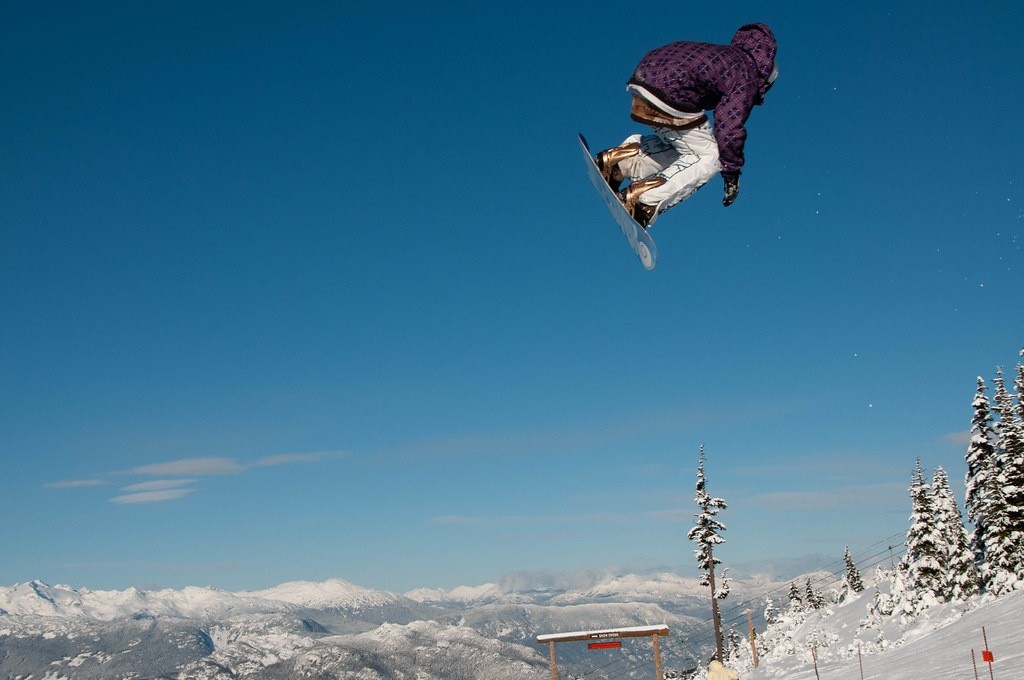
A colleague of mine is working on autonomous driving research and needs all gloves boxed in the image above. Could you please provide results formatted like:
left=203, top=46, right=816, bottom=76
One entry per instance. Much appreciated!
left=721, top=169, right=739, bottom=207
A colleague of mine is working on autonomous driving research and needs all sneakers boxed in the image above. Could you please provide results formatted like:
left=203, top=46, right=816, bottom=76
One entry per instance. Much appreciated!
left=623, top=196, right=654, bottom=232
left=605, top=150, right=626, bottom=189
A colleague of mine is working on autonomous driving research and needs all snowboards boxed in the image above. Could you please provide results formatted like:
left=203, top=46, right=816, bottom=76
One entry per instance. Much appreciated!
left=577, top=131, right=661, bottom=272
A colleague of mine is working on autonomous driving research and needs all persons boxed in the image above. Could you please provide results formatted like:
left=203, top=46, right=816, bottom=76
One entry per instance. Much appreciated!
left=594, top=22, right=778, bottom=230
